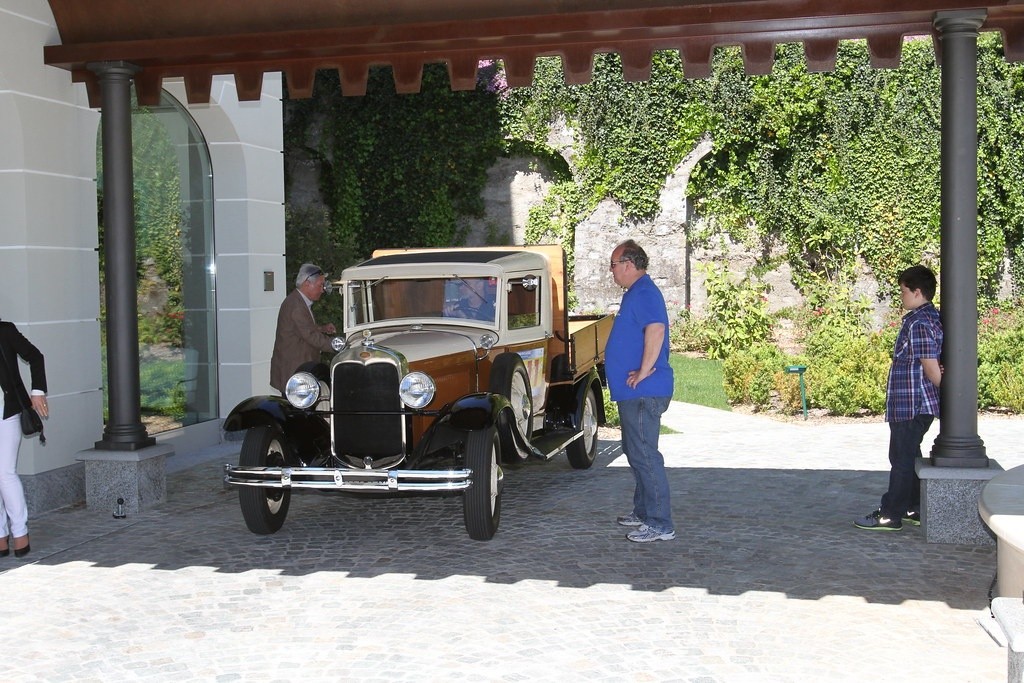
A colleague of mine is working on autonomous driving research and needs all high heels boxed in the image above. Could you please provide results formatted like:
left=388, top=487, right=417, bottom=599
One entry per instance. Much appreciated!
left=14, top=533, right=30, bottom=557
left=0, top=535, right=9, bottom=557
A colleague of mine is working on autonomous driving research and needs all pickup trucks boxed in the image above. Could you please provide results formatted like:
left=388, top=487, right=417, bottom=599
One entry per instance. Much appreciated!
left=218, top=242, right=620, bottom=543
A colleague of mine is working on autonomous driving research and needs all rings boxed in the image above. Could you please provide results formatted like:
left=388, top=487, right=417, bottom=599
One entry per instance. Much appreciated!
left=631, top=378, right=633, bottom=382
left=43, top=404, right=46, bottom=407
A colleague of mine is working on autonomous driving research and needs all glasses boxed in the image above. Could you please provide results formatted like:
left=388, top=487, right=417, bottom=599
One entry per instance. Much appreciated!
left=611, top=259, right=634, bottom=268
left=305, top=269, right=323, bottom=281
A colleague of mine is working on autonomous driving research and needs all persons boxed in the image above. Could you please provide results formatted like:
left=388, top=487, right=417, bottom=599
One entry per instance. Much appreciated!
left=270, top=263, right=339, bottom=399
left=0, top=319, right=49, bottom=558
left=440, top=277, right=495, bottom=322
left=605, top=240, right=675, bottom=543
left=853, top=265, right=944, bottom=531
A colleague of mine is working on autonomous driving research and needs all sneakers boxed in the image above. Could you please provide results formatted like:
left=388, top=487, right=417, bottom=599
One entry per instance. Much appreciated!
left=853, top=505, right=903, bottom=530
left=879, top=506, right=920, bottom=525
left=617, top=511, right=644, bottom=525
left=626, top=523, right=676, bottom=542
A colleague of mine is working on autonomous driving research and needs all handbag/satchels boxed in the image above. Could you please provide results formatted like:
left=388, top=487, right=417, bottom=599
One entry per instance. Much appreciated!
left=21, top=407, right=43, bottom=435
